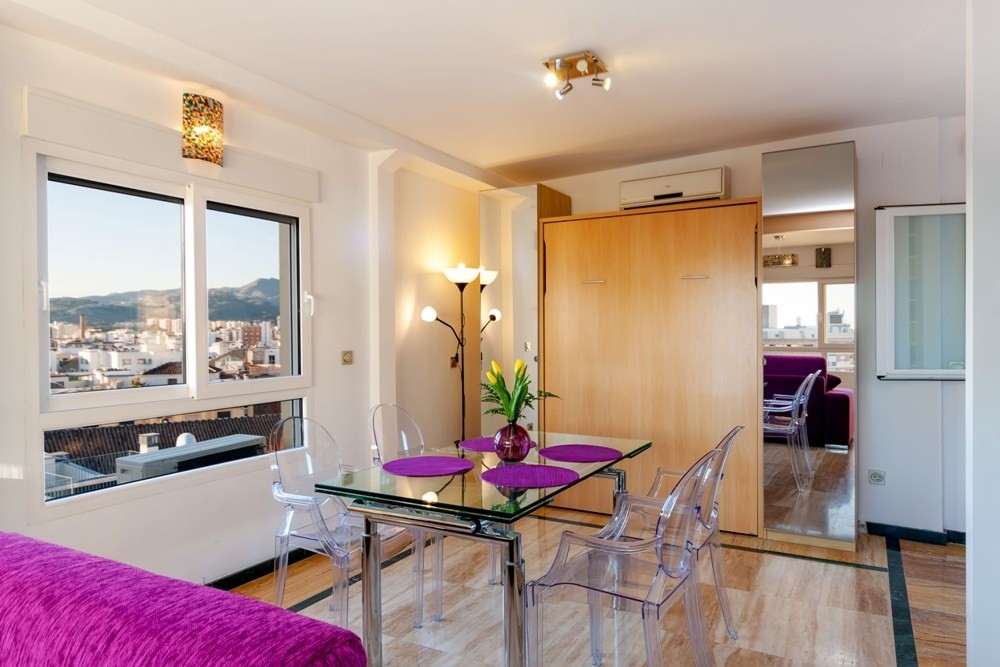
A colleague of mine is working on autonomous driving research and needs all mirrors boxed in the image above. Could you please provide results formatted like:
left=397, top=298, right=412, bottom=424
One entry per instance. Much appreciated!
left=760, top=140, right=856, bottom=546
left=482, top=451, right=540, bottom=509
left=479, top=185, right=539, bottom=430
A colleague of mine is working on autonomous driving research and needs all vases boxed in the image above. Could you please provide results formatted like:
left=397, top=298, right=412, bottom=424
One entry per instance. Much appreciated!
left=493, top=461, right=528, bottom=499
left=493, top=419, right=531, bottom=462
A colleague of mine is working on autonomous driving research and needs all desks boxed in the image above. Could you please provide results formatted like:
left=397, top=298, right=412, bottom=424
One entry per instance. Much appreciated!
left=116, top=434, right=268, bottom=485
left=44, top=449, right=144, bottom=498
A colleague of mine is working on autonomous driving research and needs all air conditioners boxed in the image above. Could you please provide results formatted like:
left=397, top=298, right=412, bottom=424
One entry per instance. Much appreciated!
left=618, top=165, right=733, bottom=211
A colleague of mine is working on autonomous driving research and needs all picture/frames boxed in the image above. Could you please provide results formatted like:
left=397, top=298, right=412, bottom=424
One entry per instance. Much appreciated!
left=872, top=202, right=966, bottom=382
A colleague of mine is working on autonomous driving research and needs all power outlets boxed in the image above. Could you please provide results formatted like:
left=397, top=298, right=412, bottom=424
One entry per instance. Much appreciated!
left=868, top=470, right=885, bottom=485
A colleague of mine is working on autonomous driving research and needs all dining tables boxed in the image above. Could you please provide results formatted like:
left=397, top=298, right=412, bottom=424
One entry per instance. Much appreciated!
left=316, top=429, right=653, bottom=667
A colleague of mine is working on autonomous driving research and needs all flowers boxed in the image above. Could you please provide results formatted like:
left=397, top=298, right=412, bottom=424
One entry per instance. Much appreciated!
left=478, top=359, right=564, bottom=422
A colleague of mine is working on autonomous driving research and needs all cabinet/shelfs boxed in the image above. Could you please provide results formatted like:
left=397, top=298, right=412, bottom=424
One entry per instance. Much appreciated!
left=760, top=140, right=856, bottom=553
left=481, top=184, right=571, bottom=431
left=539, top=195, right=763, bottom=551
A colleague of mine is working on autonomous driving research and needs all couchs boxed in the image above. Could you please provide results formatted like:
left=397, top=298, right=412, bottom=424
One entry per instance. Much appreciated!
left=0, top=532, right=368, bottom=667
left=763, top=354, right=855, bottom=449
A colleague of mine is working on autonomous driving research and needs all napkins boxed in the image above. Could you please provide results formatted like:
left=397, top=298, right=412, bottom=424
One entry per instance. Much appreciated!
left=381, top=456, right=475, bottom=476
left=459, top=436, right=536, bottom=453
left=538, top=444, right=622, bottom=463
left=480, top=464, right=580, bottom=488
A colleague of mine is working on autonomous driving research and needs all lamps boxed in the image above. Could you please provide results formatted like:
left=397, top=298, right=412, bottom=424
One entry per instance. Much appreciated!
left=421, top=444, right=466, bottom=509
left=542, top=50, right=611, bottom=99
left=479, top=270, right=502, bottom=339
left=421, top=262, right=483, bottom=440
left=182, top=93, right=224, bottom=180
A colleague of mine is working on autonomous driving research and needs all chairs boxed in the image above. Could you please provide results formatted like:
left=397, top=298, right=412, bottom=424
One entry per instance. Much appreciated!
left=763, top=368, right=822, bottom=490
left=584, top=424, right=746, bottom=655
left=523, top=446, right=723, bottom=667
left=367, top=402, right=497, bottom=622
left=269, top=415, right=429, bottom=631
